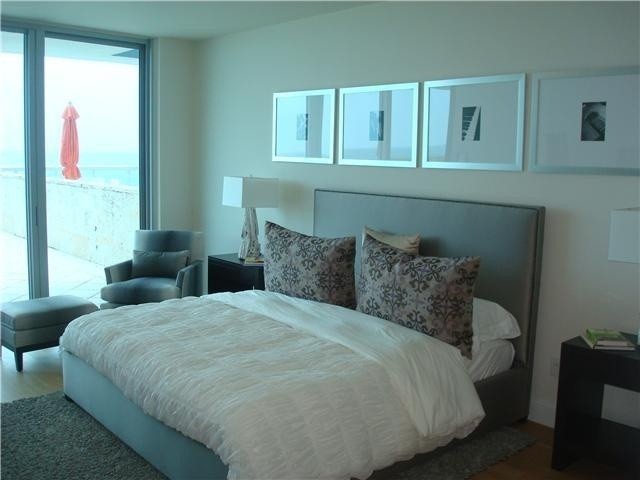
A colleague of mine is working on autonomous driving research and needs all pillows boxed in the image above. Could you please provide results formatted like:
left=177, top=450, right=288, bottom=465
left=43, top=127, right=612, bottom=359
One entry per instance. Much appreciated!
left=132, top=250, right=190, bottom=277
left=263, top=221, right=522, bottom=360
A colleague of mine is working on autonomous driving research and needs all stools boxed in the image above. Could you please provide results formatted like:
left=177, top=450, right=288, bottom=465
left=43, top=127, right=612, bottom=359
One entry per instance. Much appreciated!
left=0, top=295, right=97, bottom=372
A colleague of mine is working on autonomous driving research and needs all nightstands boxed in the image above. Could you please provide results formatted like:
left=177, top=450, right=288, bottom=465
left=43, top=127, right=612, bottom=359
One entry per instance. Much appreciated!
left=208, top=253, right=264, bottom=294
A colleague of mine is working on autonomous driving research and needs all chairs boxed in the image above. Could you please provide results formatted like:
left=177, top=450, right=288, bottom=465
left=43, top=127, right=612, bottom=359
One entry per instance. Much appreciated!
left=100, top=230, right=203, bottom=310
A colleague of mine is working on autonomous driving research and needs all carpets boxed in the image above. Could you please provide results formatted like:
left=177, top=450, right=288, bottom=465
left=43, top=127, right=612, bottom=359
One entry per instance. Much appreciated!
left=0, top=392, right=536, bottom=480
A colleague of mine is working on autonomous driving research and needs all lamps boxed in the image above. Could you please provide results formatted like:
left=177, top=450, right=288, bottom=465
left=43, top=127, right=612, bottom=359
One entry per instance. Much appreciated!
left=608, top=207, right=640, bottom=264
left=222, top=176, right=279, bottom=260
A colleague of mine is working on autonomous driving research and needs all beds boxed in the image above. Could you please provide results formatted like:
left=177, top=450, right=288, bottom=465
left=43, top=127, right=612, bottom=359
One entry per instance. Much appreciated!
left=62, top=190, right=545, bottom=480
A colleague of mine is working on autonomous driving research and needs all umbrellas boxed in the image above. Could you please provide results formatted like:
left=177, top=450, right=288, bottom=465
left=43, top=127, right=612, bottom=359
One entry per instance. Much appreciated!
left=59, top=101, right=81, bottom=180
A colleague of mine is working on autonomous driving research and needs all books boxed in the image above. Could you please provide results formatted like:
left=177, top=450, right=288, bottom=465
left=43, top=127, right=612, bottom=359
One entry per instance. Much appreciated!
left=243, top=256, right=264, bottom=268
left=578, top=328, right=634, bottom=351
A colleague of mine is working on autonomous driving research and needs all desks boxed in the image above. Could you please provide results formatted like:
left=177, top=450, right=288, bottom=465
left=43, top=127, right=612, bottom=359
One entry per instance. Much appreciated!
left=552, top=337, right=640, bottom=471
